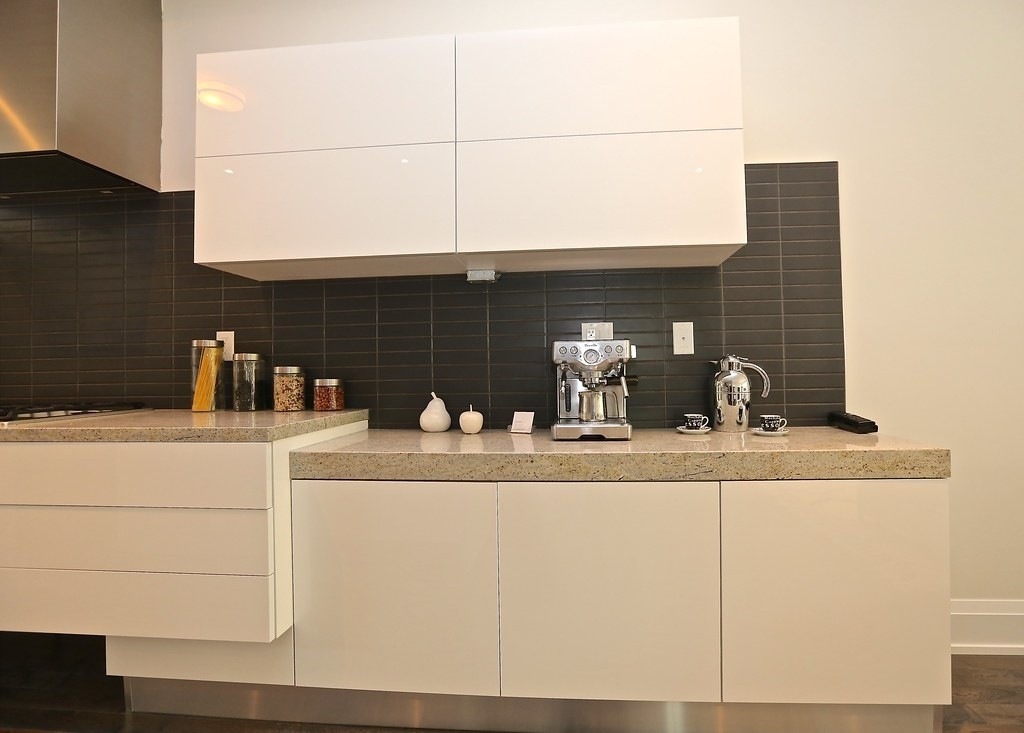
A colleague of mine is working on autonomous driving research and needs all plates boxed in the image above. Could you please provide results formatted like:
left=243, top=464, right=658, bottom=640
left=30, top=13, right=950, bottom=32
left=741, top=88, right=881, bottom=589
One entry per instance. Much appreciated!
left=751, top=428, right=789, bottom=436
left=677, top=426, right=712, bottom=434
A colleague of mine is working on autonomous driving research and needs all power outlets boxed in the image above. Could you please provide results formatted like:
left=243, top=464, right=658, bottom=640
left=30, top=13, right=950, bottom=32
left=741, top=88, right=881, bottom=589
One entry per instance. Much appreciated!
left=582, top=322, right=613, bottom=340
left=216, top=330, right=234, bottom=362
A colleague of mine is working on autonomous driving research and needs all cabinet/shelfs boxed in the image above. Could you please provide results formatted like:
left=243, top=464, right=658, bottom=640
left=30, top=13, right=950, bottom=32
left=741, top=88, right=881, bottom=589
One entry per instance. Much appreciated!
left=193, top=11, right=748, bottom=281
left=0, top=439, right=955, bottom=733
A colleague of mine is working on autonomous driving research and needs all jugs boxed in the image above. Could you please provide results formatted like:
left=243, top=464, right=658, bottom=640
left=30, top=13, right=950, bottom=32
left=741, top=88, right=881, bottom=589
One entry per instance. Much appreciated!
left=709, top=352, right=770, bottom=432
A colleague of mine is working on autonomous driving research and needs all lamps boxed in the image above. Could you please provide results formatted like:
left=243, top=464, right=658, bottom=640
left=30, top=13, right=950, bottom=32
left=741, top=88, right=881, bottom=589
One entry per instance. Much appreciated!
left=196, top=80, right=248, bottom=114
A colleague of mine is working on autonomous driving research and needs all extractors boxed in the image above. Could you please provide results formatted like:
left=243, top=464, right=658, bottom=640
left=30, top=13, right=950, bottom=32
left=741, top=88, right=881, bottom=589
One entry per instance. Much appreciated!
left=0, top=0, right=162, bottom=207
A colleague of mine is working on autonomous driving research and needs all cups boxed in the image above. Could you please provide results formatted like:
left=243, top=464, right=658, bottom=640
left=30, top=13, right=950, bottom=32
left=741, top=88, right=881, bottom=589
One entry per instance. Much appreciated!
left=760, top=415, right=787, bottom=432
left=683, top=413, right=709, bottom=430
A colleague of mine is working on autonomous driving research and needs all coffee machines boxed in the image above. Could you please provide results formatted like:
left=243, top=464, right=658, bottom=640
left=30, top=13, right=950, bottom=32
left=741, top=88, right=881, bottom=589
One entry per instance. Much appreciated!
left=552, top=339, right=632, bottom=440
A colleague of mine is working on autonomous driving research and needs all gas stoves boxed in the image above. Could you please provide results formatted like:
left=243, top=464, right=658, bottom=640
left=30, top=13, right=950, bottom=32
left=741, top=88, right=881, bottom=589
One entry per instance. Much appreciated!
left=0, top=402, right=144, bottom=421
